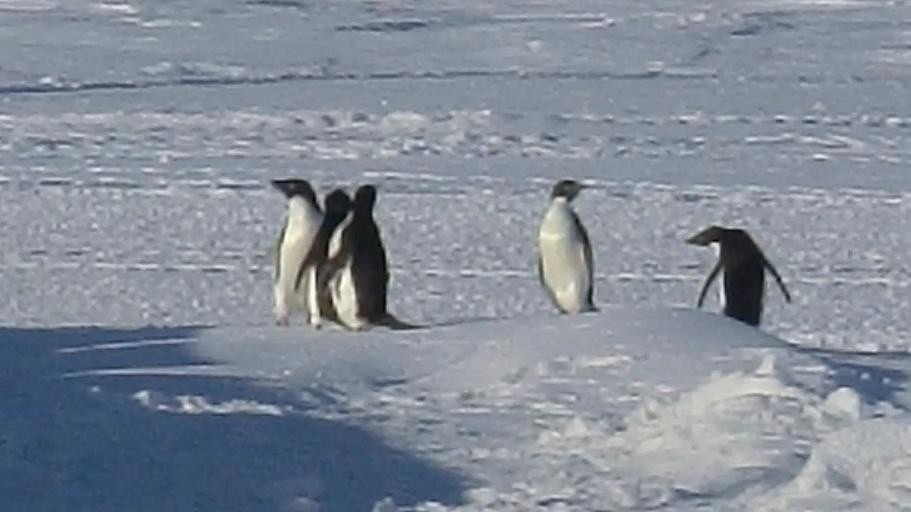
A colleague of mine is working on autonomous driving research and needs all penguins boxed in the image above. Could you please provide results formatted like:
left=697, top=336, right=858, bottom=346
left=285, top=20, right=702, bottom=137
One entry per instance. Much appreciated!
left=268, top=177, right=406, bottom=329
left=684, top=224, right=793, bottom=330
left=535, top=180, right=606, bottom=316
